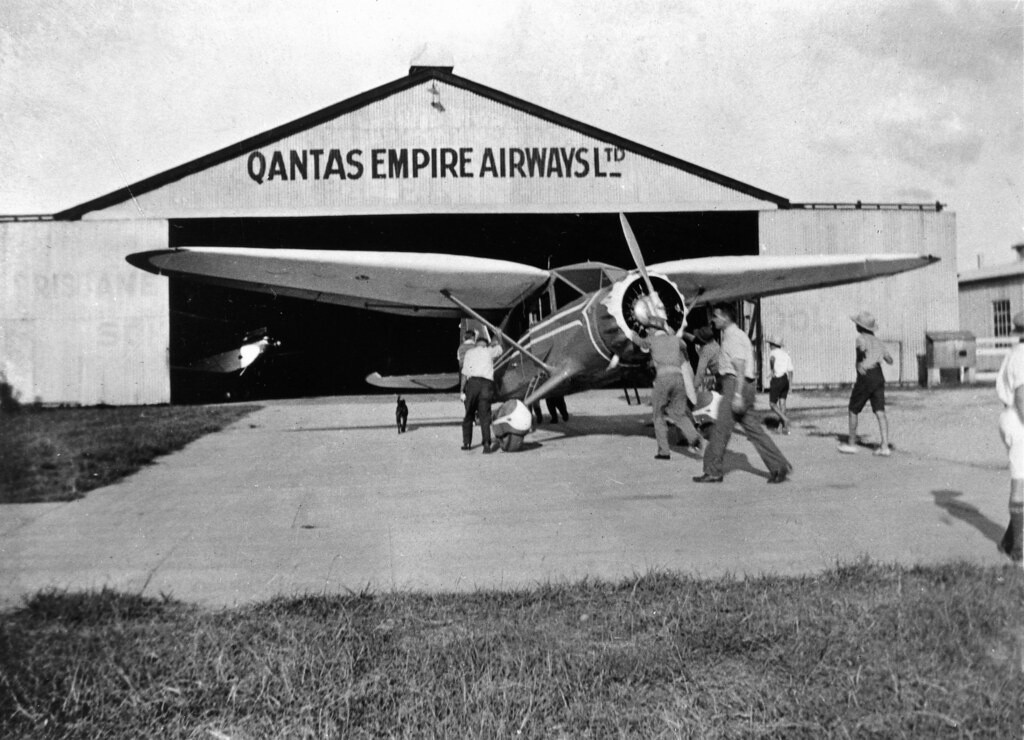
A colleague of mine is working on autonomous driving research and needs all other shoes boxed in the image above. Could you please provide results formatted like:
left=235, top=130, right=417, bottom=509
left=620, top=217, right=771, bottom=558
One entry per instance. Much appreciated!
left=767, top=463, right=793, bottom=484
left=775, top=421, right=792, bottom=436
left=872, top=447, right=891, bottom=456
left=692, top=474, right=723, bottom=484
left=461, top=443, right=471, bottom=451
left=690, top=437, right=703, bottom=451
left=838, top=443, right=859, bottom=454
left=654, top=453, right=670, bottom=459
left=483, top=445, right=490, bottom=453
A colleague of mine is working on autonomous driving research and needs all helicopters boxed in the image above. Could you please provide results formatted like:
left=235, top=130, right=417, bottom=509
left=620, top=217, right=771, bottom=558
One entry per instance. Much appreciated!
left=124, top=213, right=944, bottom=452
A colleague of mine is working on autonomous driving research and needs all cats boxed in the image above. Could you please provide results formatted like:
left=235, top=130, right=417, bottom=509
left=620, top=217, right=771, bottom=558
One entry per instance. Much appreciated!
left=396, top=395, right=408, bottom=434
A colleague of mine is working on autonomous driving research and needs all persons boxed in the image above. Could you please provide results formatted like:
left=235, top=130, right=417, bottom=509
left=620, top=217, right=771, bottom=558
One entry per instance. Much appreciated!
left=633, top=316, right=703, bottom=460
left=995, top=310, right=1024, bottom=563
left=528, top=400, right=543, bottom=432
left=764, top=333, right=793, bottom=436
left=460, top=337, right=503, bottom=453
left=683, top=327, right=726, bottom=396
left=837, top=310, right=892, bottom=456
left=692, top=300, right=793, bottom=484
left=545, top=396, right=569, bottom=425
left=457, top=330, right=481, bottom=425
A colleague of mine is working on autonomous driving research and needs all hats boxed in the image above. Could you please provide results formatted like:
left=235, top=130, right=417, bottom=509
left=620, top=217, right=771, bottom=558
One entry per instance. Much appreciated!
left=765, top=335, right=784, bottom=347
left=850, top=311, right=878, bottom=332
left=643, top=316, right=666, bottom=330
left=693, top=326, right=714, bottom=344
left=1009, top=310, right=1024, bottom=339
